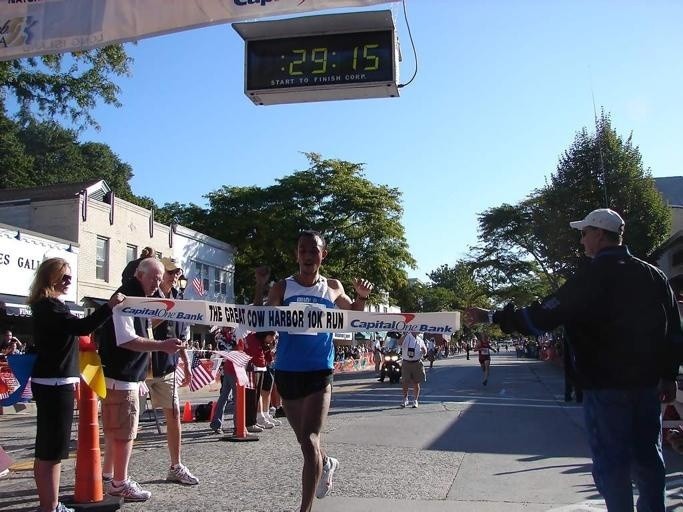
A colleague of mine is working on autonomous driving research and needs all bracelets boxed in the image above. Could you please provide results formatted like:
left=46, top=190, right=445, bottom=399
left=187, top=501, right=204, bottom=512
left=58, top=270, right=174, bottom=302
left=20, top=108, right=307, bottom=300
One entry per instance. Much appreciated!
left=488, top=309, right=496, bottom=324
left=357, top=294, right=370, bottom=301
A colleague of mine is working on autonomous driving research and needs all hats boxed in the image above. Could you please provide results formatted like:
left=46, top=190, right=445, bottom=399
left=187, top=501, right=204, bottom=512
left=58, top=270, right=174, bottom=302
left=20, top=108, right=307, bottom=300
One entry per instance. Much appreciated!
left=569, top=209, right=625, bottom=236
left=160, top=257, right=183, bottom=275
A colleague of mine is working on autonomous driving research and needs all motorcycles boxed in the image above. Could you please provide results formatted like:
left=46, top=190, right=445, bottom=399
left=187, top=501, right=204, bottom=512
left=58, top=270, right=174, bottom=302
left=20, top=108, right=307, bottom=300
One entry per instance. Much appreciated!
left=376, top=347, right=403, bottom=384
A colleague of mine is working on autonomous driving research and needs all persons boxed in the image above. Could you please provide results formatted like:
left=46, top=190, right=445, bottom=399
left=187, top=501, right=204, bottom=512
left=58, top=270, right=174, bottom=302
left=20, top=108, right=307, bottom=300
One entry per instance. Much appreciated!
left=122, top=246, right=157, bottom=284
left=29, top=257, right=126, bottom=512
left=254, top=230, right=374, bottom=512
left=1, top=330, right=26, bottom=357
left=334, top=331, right=565, bottom=386
left=467, top=207, right=683, bottom=511
left=93, top=258, right=187, bottom=501
left=205, top=327, right=282, bottom=434
left=181, top=335, right=212, bottom=359
left=398, top=332, right=428, bottom=408
left=101, top=254, right=200, bottom=486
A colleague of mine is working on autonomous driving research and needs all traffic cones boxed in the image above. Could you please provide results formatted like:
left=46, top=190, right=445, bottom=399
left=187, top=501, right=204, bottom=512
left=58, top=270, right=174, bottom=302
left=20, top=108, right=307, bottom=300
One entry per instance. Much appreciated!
left=54, top=342, right=124, bottom=511
left=661, top=405, right=682, bottom=446
left=208, top=400, right=217, bottom=423
left=180, top=401, right=195, bottom=423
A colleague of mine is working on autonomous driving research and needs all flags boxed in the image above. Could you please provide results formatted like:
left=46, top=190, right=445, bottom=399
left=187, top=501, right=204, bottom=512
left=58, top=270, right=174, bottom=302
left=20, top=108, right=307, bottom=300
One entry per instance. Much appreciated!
left=188, top=354, right=214, bottom=392
left=193, top=272, right=206, bottom=296
left=215, top=350, right=252, bottom=366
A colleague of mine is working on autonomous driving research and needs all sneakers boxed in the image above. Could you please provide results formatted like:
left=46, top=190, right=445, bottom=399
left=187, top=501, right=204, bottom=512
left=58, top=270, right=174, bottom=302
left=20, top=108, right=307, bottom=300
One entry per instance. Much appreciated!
left=315, top=457, right=339, bottom=498
left=412, top=400, right=418, bottom=407
left=37, top=407, right=286, bottom=512
left=400, top=398, right=409, bottom=407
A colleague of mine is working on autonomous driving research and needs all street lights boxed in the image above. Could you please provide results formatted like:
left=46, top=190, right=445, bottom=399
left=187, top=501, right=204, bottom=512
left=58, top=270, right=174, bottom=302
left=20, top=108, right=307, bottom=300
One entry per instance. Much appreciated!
left=177, top=275, right=187, bottom=301
